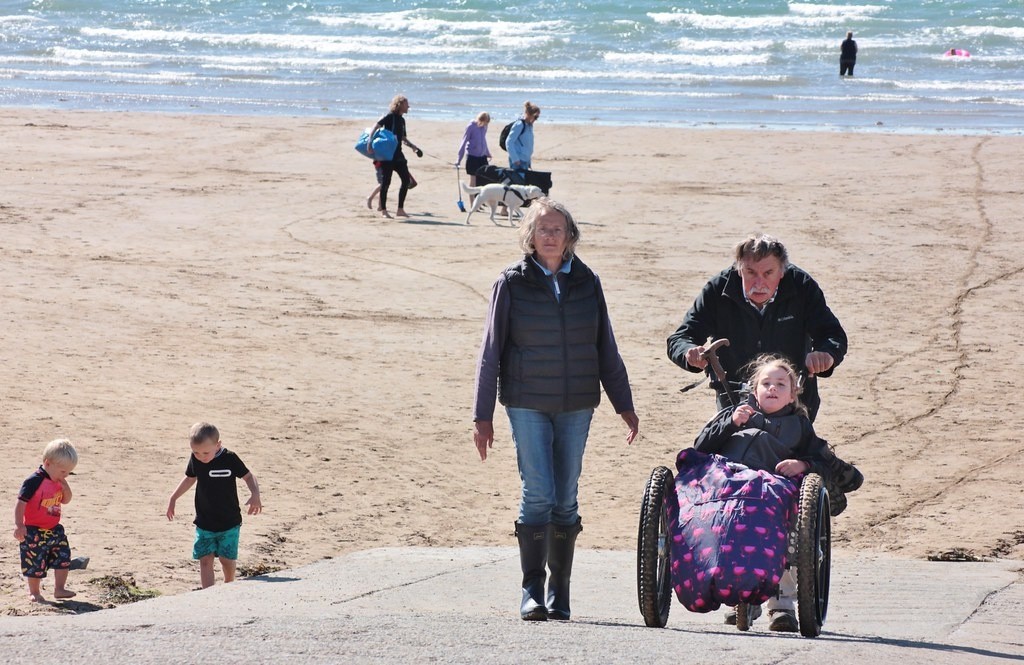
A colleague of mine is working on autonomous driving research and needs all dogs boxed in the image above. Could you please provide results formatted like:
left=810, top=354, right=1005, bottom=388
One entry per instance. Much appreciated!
left=461, top=182, right=545, bottom=226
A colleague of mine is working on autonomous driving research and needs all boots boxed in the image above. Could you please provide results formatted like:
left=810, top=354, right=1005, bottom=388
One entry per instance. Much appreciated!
left=547, top=514, right=583, bottom=619
left=514, top=519, right=550, bottom=621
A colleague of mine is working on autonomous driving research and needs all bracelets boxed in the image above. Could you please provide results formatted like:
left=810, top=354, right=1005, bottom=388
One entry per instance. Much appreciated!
left=368, top=140, right=372, bottom=142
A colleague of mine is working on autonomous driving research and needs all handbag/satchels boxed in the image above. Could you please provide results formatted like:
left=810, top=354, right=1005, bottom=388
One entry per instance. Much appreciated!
left=355, top=112, right=398, bottom=161
left=475, top=165, right=552, bottom=206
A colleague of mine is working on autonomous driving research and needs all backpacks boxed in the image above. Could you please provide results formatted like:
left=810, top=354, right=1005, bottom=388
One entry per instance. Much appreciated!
left=499, top=118, right=525, bottom=152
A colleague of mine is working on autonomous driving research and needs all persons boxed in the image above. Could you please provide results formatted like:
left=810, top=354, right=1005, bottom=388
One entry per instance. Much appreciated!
left=693, top=356, right=832, bottom=478
left=472, top=197, right=639, bottom=620
left=367, top=96, right=423, bottom=218
left=455, top=112, right=491, bottom=211
left=667, top=234, right=848, bottom=632
left=13, top=439, right=78, bottom=602
left=166, top=422, right=262, bottom=589
left=839, top=31, right=858, bottom=76
left=500, top=102, right=540, bottom=215
left=367, top=160, right=383, bottom=211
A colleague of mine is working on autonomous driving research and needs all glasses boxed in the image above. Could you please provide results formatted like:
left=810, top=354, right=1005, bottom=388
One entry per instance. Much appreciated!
left=533, top=114, right=539, bottom=120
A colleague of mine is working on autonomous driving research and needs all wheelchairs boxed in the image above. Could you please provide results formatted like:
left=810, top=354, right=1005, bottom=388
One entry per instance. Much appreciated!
left=632, top=337, right=834, bottom=640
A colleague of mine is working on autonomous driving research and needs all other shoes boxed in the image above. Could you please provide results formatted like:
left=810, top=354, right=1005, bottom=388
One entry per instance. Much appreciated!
left=768, top=610, right=799, bottom=632
left=725, top=604, right=763, bottom=625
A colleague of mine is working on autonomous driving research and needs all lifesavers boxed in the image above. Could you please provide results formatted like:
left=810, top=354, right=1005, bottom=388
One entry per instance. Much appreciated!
left=943, top=47, right=970, bottom=57
